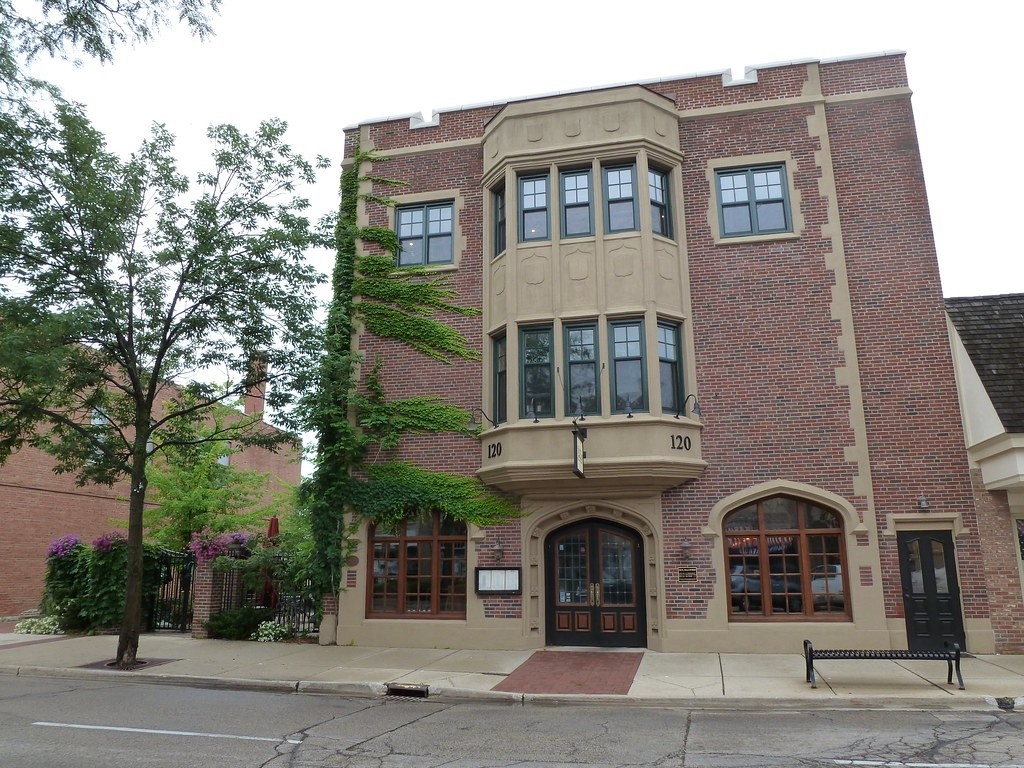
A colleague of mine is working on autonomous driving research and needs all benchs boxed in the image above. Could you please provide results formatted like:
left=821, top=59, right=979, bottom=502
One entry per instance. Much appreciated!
left=803, top=640, right=966, bottom=690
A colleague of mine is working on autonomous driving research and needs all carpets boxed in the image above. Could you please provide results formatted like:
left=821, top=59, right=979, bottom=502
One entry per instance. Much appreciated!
left=487, top=651, right=646, bottom=695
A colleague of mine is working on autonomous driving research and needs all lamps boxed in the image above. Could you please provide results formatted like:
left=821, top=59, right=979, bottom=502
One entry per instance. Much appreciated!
left=468, top=394, right=703, bottom=429
left=681, top=537, right=694, bottom=560
left=492, top=537, right=504, bottom=562
left=917, top=492, right=929, bottom=510
